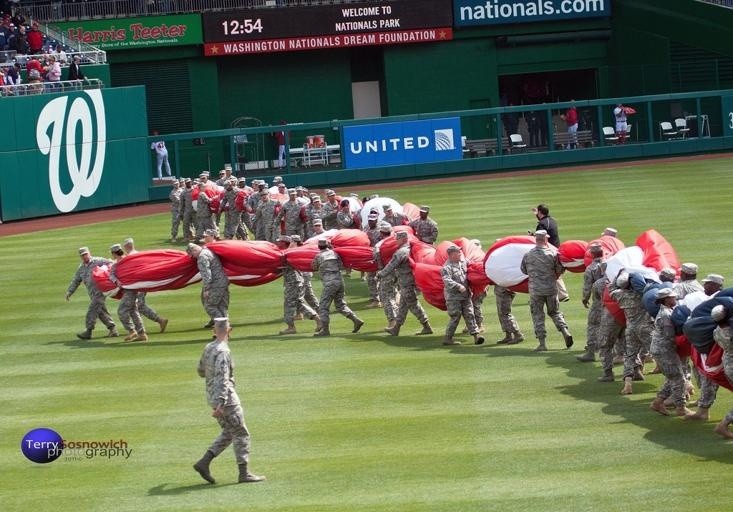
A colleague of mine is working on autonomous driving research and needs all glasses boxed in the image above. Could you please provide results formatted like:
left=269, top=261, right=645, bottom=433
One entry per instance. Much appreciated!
left=219, top=172, right=225, bottom=175
left=278, top=186, right=286, bottom=189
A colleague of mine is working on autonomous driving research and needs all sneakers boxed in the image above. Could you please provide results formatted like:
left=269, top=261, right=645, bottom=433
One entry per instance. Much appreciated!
left=559, top=295, right=569, bottom=302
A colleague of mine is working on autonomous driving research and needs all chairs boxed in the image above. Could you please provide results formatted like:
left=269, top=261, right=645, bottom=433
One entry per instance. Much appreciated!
left=660, top=121, right=678, bottom=140
left=623, top=125, right=632, bottom=144
left=510, top=134, right=527, bottom=155
left=602, top=126, right=620, bottom=147
left=675, top=118, right=690, bottom=140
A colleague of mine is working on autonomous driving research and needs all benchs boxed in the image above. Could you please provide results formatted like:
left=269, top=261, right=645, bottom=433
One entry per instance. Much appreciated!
left=465, top=137, right=512, bottom=157
left=553, top=130, right=593, bottom=150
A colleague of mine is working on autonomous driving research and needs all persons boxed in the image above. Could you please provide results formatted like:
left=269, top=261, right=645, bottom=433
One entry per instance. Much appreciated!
left=186, top=240, right=231, bottom=338
left=364, top=214, right=393, bottom=309
left=409, top=207, right=438, bottom=245
left=373, top=228, right=436, bottom=337
left=582, top=223, right=733, bottom=442
left=500, top=101, right=523, bottom=152
left=289, top=233, right=321, bottom=323
left=311, top=239, right=365, bottom=338
left=528, top=202, right=570, bottom=301
left=441, top=243, right=484, bottom=347
left=526, top=101, right=543, bottom=148
left=109, top=244, right=149, bottom=344
left=188, top=317, right=267, bottom=485
left=519, top=230, right=576, bottom=351
left=64, top=246, right=121, bottom=341
left=381, top=203, right=410, bottom=229
left=1, top=1, right=82, bottom=95
left=171, top=167, right=375, bottom=245
left=276, top=234, right=322, bottom=336
left=559, top=95, right=585, bottom=151
left=373, top=220, right=403, bottom=333
left=123, top=237, right=169, bottom=335
left=269, top=120, right=293, bottom=169
left=614, top=97, right=632, bottom=148
left=493, top=237, right=526, bottom=346
left=149, top=131, right=173, bottom=178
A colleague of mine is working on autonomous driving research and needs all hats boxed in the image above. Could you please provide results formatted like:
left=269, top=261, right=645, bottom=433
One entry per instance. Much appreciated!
left=226, top=167, right=233, bottom=171
left=657, top=289, right=677, bottom=299
left=702, top=274, right=726, bottom=287
left=172, top=180, right=180, bottom=185
left=287, top=189, right=298, bottom=195
left=617, top=272, right=629, bottom=288
left=256, top=180, right=265, bottom=185
left=534, top=229, right=550, bottom=238
left=471, top=239, right=482, bottom=249
left=446, top=246, right=460, bottom=253
left=326, top=190, right=336, bottom=197
left=198, top=182, right=207, bottom=189
left=79, top=247, right=89, bottom=255
left=318, top=237, right=327, bottom=246
left=312, top=218, right=322, bottom=226
left=310, top=192, right=316, bottom=197
left=200, top=174, right=206, bottom=177
left=180, top=178, right=185, bottom=182
left=212, top=318, right=230, bottom=328
left=110, top=244, right=122, bottom=252
left=370, top=194, right=378, bottom=199
left=495, top=239, right=501, bottom=243
left=312, top=195, right=322, bottom=203
left=351, top=193, right=359, bottom=200
left=659, top=268, right=677, bottom=280
left=203, top=171, right=209, bottom=176
left=184, top=178, right=191, bottom=183
left=680, top=262, right=698, bottom=275
left=711, top=305, right=725, bottom=322
left=601, top=227, right=618, bottom=239
left=230, top=178, right=236, bottom=182
left=259, top=183, right=268, bottom=188
left=239, top=177, right=245, bottom=183
left=296, top=186, right=302, bottom=191
left=259, top=189, right=269, bottom=195
left=291, top=235, right=301, bottom=241
left=370, top=207, right=380, bottom=213
left=253, top=180, right=257, bottom=185
left=123, top=238, right=134, bottom=247
left=194, top=179, right=200, bottom=183
left=219, top=170, right=226, bottom=174
left=396, top=231, right=408, bottom=240
left=367, top=214, right=379, bottom=221
left=382, top=203, right=392, bottom=212
left=303, top=188, right=308, bottom=192
left=419, top=206, right=430, bottom=213
left=223, top=180, right=231, bottom=190
left=379, top=221, right=391, bottom=232
left=589, top=244, right=604, bottom=253
left=273, top=177, right=282, bottom=182
left=276, top=236, right=290, bottom=243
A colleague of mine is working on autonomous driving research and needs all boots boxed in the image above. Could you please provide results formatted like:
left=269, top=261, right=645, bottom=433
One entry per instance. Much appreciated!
left=182, top=237, right=188, bottom=242
left=686, top=405, right=710, bottom=421
left=612, top=354, right=625, bottom=364
left=293, top=311, right=304, bottom=321
left=560, top=327, right=575, bottom=349
left=125, top=329, right=138, bottom=341
left=474, top=333, right=486, bottom=345
left=479, top=326, right=485, bottom=333
left=349, top=314, right=365, bottom=333
left=665, top=396, right=681, bottom=405
left=685, top=380, right=694, bottom=393
left=716, top=418, right=732, bottom=439
left=443, top=335, right=461, bottom=345
left=463, top=326, right=468, bottom=333
left=171, top=234, right=176, bottom=243
left=684, top=393, right=690, bottom=399
left=77, top=330, right=92, bottom=340
left=497, top=332, right=513, bottom=344
left=649, top=398, right=670, bottom=416
left=346, top=269, right=352, bottom=277
left=509, top=331, right=524, bottom=345
left=193, top=450, right=222, bottom=486
left=366, top=299, right=381, bottom=308
left=106, top=327, right=118, bottom=337
left=634, top=367, right=644, bottom=379
left=416, top=321, right=434, bottom=335
left=644, top=355, right=654, bottom=362
left=577, top=350, right=596, bottom=363
left=676, top=406, right=695, bottom=416
left=599, top=371, right=614, bottom=381
left=313, top=315, right=324, bottom=331
left=620, top=376, right=633, bottom=395
left=395, top=293, right=401, bottom=305
left=134, top=333, right=147, bottom=342
left=360, top=272, right=365, bottom=281
left=533, top=338, right=548, bottom=352
left=159, top=318, right=169, bottom=333
left=649, top=366, right=661, bottom=374
left=384, top=322, right=402, bottom=336
left=194, top=237, right=201, bottom=243
left=388, top=320, right=395, bottom=327
left=236, top=462, right=268, bottom=484
left=313, top=322, right=331, bottom=337
left=280, top=322, right=297, bottom=334
